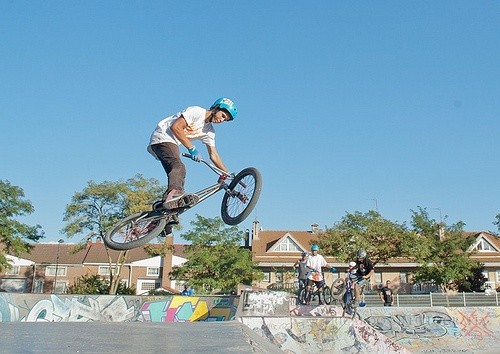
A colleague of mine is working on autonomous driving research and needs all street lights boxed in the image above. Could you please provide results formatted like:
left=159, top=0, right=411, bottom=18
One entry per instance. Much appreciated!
left=53, top=238, right=65, bottom=294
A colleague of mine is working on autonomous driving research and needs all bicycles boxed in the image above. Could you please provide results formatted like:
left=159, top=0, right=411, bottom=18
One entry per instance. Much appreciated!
left=297, top=268, right=333, bottom=306
left=104, top=153, right=263, bottom=251
left=331, top=268, right=365, bottom=320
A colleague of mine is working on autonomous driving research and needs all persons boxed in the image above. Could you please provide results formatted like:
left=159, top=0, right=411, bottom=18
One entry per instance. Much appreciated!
left=146, top=97, right=239, bottom=235
left=346, top=248, right=375, bottom=307
left=293, top=245, right=336, bottom=305
left=379, top=280, right=395, bottom=306
